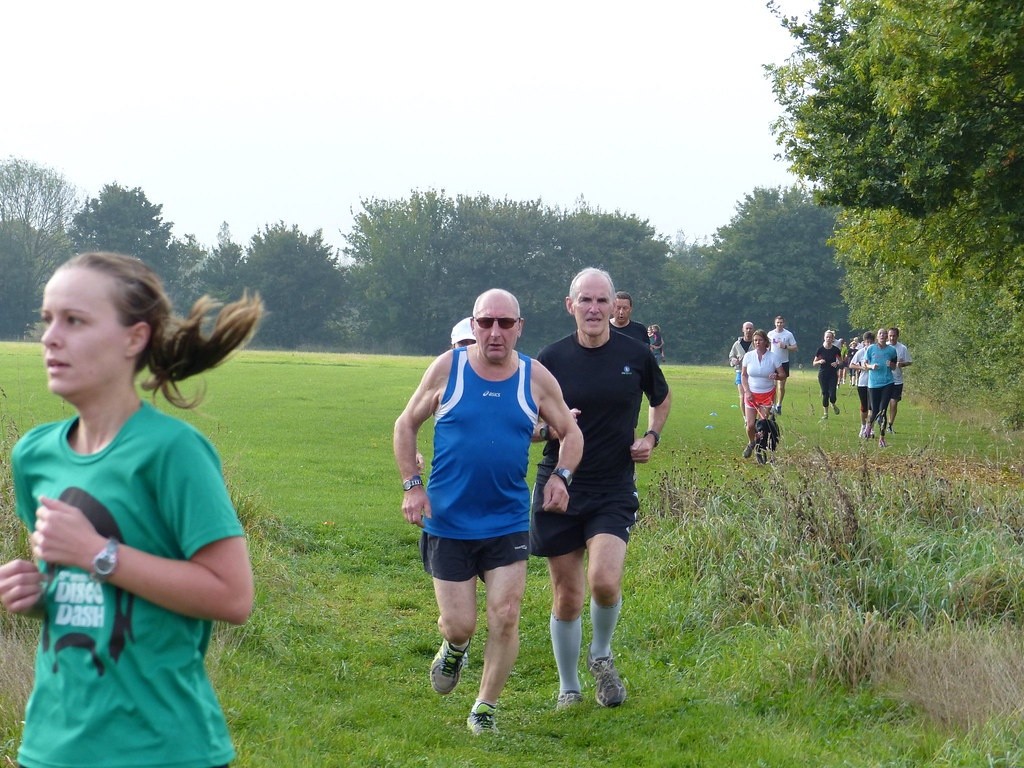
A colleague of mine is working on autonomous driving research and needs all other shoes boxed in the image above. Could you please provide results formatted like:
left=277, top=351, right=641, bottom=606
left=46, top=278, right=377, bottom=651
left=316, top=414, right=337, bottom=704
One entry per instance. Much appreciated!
left=879, top=440, right=886, bottom=448
left=864, top=425, right=870, bottom=438
left=743, top=422, right=747, bottom=427
left=889, top=426, right=892, bottom=432
left=822, top=414, right=828, bottom=419
left=776, top=405, right=781, bottom=414
left=859, top=427, right=865, bottom=437
left=871, top=428, right=875, bottom=435
left=831, top=405, right=839, bottom=414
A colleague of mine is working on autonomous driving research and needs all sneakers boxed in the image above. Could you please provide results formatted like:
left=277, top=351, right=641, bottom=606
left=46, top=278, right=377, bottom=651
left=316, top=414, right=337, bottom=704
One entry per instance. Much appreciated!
left=743, top=442, right=755, bottom=457
left=586, top=643, right=626, bottom=707
left=430, top=636, right=471, bottom=694
left=557, top=690, right=582, bottom=711
left=467, top=702, right=500, bottom=736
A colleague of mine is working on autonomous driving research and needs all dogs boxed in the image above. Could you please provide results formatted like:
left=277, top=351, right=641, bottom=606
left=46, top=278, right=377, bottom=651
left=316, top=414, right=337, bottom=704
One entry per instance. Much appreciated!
left=755, top=412, right=781, bottom=463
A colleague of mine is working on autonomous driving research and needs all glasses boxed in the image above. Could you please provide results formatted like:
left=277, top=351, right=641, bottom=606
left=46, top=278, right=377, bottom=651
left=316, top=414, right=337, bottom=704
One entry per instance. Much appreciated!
left=472, top=316, right=521, bottom=329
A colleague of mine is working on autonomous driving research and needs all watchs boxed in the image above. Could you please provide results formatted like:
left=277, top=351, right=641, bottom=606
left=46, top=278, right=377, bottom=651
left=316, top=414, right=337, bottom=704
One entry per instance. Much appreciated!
left=403, top=478, right=422, bottom=491
left=89, top=536, right=120, bottom=584
left=540, top=424, right=550, bottom=441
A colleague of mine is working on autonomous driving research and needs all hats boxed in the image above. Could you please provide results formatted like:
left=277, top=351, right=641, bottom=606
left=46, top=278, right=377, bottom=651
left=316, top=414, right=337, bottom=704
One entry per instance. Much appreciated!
left=451, top=317, right=477, bottom=344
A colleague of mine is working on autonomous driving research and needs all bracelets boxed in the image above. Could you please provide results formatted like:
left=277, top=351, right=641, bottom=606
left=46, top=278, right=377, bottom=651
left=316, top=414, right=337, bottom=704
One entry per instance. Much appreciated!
left=783, top=345, right=787, bottom=349
left=552, top=468, right=573, bottom=486
left=403, top=475, right=420, bottom=483
left=644, top=430, right=661, bottom=447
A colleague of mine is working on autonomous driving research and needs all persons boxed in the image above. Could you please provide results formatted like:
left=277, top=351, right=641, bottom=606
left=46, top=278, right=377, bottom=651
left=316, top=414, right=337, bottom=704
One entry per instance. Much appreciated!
left=741, top=329, right=787, bottom=462
left=646, top=324, right=666, bottom=367
left=530, top=266, right=672, bottom=709
left=729, top=322, right=757, bottom=425
left=608, top=289, right=650, bottom=345
left=813, top=328, right=847, bottom=419
left=394, top=288, right=584, bottom=735
left=1, top=250, right=268, bottom=768
left=846, top=328, right=913, bottom=447
left=767, top=315, right=799, bottom=415
left=449, top=319, right=489, bottom=670
